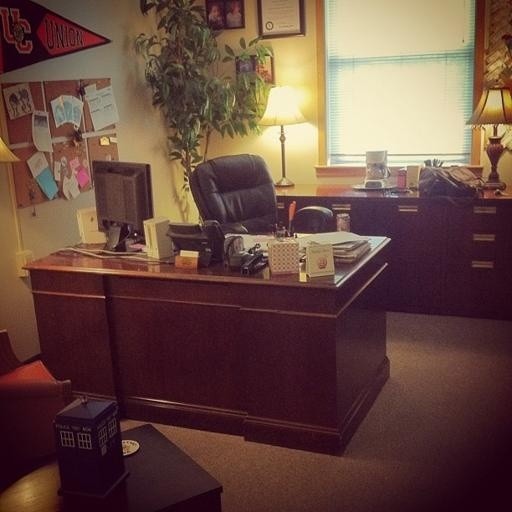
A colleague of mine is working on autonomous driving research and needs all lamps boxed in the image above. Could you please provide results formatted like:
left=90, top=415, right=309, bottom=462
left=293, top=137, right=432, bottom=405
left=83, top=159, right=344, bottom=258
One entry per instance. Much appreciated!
left=466, top=87, right=512, bottom=190
left=257, top=85, right=310, bottom=188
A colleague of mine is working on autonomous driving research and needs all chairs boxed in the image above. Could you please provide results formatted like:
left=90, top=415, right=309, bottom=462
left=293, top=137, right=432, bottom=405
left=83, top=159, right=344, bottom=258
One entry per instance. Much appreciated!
left=0, top=329, right=74, bottom=480
left=188, top=154, right=334, bottom=239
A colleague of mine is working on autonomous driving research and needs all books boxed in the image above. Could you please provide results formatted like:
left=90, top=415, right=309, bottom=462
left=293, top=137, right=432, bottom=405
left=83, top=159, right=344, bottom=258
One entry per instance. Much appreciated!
left=294, top=230, right=373, bottom=263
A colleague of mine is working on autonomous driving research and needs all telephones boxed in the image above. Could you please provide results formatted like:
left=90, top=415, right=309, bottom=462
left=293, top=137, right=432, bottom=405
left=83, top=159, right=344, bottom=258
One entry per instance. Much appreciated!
left=167, top=219, right=227, bottom=266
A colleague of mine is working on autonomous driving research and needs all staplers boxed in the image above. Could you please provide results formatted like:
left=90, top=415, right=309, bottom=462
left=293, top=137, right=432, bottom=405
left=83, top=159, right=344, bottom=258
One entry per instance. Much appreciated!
left=240, top=253, right=267, bottom=275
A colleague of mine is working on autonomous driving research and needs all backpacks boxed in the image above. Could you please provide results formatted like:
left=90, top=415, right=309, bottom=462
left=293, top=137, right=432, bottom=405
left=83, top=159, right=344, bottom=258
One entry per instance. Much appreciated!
left=419, top=167, right=484, bottom=196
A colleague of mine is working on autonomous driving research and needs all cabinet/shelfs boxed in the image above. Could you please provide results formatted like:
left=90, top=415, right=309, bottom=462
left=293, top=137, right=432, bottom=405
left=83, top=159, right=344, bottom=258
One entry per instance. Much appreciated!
left=263, top=184, right=512, bottom=323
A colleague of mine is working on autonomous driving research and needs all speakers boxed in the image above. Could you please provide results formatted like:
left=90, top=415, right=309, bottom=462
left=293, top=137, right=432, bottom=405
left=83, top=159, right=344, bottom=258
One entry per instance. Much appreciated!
left=142, top=216, right=174, bottom=260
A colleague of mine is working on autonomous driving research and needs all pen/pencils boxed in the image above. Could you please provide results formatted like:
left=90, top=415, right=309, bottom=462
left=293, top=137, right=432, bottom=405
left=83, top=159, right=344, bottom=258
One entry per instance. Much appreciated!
left=288, top=201, right=298, bottom=237
left=424, top=159, right=444, bottom=167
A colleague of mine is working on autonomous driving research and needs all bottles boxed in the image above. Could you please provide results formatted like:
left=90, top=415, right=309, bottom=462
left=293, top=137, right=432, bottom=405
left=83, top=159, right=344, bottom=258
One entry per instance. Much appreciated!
left=396, top=168, right=407, bottom=193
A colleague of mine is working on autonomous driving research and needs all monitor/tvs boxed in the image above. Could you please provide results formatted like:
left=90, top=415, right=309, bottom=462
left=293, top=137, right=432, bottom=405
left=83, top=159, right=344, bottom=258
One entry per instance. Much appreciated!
left=92, top=160, right=154, bottom=257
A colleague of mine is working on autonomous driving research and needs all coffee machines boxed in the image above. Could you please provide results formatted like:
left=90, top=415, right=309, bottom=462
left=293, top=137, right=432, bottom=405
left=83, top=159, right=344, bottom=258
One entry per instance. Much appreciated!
left=363, top=150, right=392, bottom=189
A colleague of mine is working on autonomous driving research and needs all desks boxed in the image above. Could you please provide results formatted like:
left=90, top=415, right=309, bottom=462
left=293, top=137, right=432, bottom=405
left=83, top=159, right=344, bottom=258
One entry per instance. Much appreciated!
left=22, top=233, right=392, bottom=458
left=0, top=423, right=228, bottom=512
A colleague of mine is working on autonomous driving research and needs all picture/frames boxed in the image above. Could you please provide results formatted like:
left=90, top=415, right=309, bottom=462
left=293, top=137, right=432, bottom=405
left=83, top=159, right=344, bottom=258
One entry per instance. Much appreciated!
left=235, top=55, right=275, bottom=88
left=257, top=0, right=306, bottom=40
left=206, top=0, right=246, bottom=30
